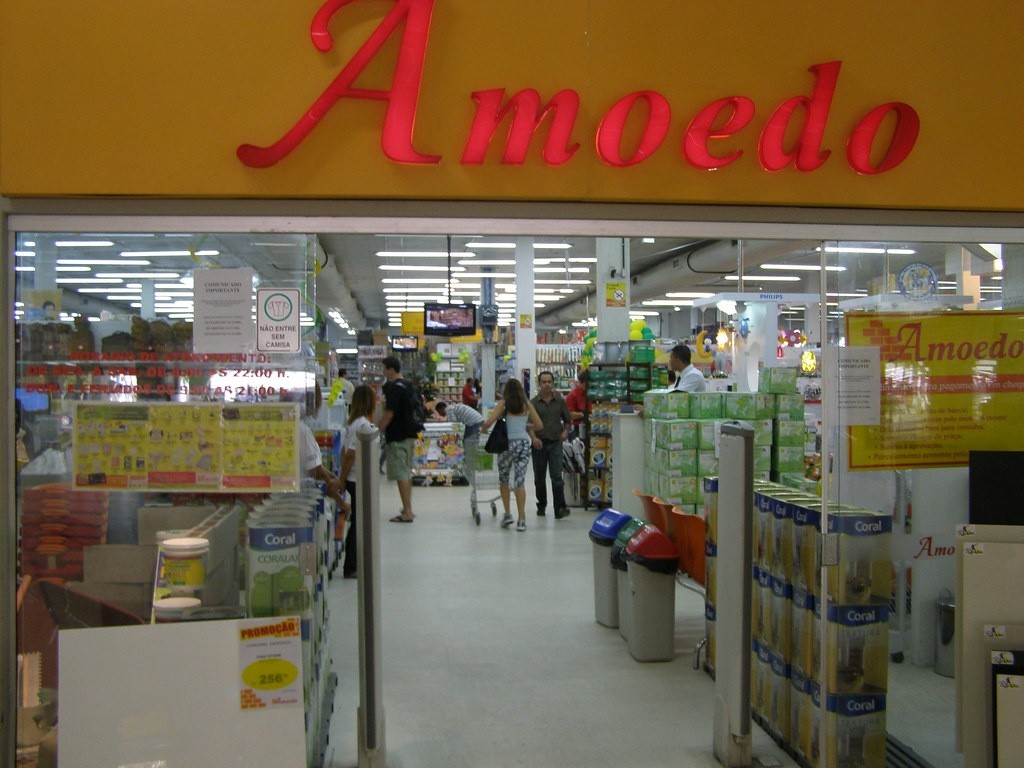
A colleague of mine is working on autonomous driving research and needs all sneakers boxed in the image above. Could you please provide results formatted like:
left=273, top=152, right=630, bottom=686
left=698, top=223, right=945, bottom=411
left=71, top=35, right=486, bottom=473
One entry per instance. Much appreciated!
left=501, top=514, right=513, bottom=528
left=515, top=520, right=527, bottom=531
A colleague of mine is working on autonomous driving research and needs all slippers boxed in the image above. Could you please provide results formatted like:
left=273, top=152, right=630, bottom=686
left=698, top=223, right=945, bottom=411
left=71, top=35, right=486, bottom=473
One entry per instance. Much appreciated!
left=389, top=516, right=413, bottom=522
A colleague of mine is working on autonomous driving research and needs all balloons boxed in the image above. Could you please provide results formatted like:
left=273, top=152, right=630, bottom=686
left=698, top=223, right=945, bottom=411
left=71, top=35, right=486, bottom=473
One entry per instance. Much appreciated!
left=582, top=320, right=657, bottom=370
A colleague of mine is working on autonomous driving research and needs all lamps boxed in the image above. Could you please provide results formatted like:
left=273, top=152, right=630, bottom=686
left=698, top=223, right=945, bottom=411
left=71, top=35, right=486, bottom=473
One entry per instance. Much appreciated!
left=367, top=237, right=922, bottom=330
left=15, top=240, right=315, bottom=329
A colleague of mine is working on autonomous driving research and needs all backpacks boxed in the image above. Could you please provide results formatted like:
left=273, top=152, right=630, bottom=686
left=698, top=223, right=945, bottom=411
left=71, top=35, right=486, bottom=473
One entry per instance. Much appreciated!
left=396, top=382, right=427, bottom=428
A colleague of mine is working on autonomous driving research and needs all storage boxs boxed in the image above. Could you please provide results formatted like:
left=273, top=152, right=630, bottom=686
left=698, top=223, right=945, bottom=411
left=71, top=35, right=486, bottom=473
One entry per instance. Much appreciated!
left=451, top=362, right=465, bottom=366
left=452, top=342, right=467, bottom=355
left=631, top=345, right=657, bottom=363
left=451, top=367, right=466, bottom=371
left=561, top=376, right=574, bottom=388
left=435, top=342, right=452, bottom=355
left=450, top=359, right=459, bottom=362
left=589, top=362, right=894, bottom=768
left=435, top=359, right=451, bottom=371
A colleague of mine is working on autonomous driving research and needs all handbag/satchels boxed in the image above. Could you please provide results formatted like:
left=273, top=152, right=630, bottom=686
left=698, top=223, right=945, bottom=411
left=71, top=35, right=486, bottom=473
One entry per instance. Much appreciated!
left=484, top=418, right=508, bottom=454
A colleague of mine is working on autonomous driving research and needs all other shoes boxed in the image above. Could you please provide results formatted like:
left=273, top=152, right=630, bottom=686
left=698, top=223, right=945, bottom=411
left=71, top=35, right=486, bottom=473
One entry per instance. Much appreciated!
left=537, top=508, right=546, bottom=516
left=555, top=509, right=570, bottom=519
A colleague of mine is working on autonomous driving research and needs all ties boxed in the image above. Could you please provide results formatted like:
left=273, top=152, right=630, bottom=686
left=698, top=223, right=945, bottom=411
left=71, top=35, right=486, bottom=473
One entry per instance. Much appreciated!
left=674, top=376, right=681, bottom=388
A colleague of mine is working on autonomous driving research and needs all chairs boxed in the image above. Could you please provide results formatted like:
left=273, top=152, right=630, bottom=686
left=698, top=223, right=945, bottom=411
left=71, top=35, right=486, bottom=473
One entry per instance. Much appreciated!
left=673, top=506, right=706, bottom=586
left=630, top=487, right=653, bottom=523
left=650, top=496, right=685, bottom=574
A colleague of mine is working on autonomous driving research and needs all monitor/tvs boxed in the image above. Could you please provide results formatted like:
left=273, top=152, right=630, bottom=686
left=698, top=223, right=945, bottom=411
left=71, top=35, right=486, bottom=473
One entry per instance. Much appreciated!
left=14, top=384, right=51, bottom=415
left=423, top=303, right=477, bottom=337
left=392, top=335, right=418, bottom=352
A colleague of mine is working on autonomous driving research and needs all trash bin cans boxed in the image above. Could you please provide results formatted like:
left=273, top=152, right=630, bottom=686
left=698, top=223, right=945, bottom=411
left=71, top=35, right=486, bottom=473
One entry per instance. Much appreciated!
left=624, top=524, right=680, bottom=662
left=588, top=510, right=632, bottom=628
left=613, top=518, right=647, bottom=638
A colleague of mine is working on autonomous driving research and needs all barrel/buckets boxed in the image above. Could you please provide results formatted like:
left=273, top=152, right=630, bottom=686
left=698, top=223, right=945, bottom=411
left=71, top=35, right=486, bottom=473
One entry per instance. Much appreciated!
left=162, top=538, right=210, bottom=591
left=153, top=597, right=202, bottom=623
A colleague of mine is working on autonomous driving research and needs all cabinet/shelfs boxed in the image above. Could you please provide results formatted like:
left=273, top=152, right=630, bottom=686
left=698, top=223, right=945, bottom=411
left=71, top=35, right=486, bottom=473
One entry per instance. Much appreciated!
left=496, top=352, right=508, bottom=399
left=437, top=343, right=465, bottom=402
left=536, top=344, right=582, bottom=391
left=356, top=346, right=387, bottom=388
left=583, top=364, right=652, bottom=510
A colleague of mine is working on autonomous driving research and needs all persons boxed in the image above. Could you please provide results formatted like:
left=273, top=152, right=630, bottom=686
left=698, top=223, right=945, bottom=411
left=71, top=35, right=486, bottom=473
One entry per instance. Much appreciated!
left=661, top=344, right=710, bottom=394
left=40, top=301, right=56, bottom=322
left=297, top=357, right=594, bottom=579
